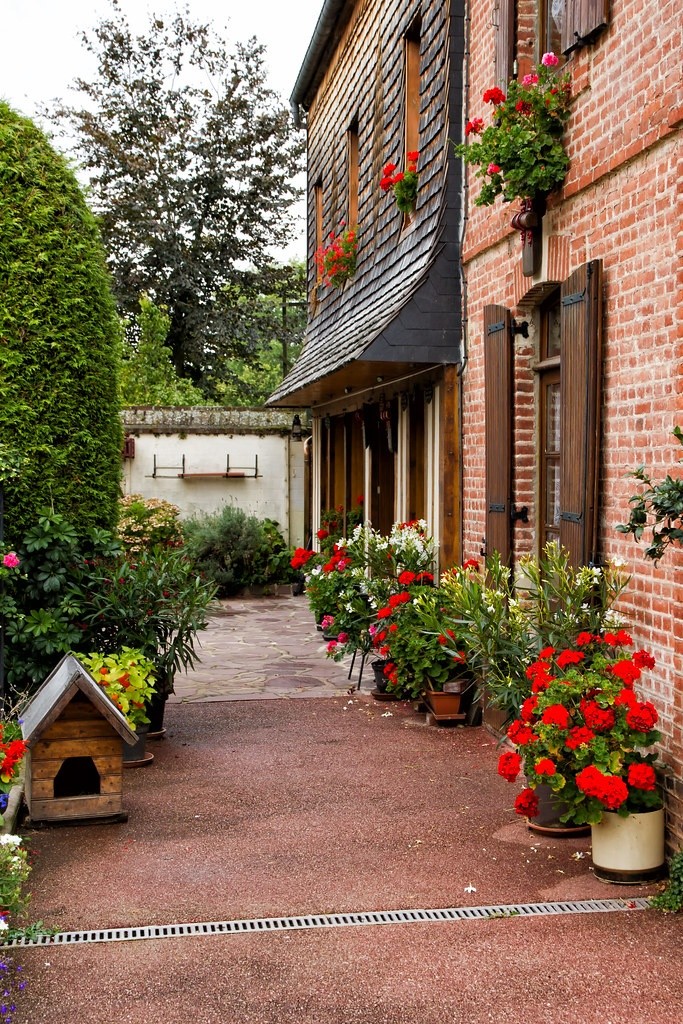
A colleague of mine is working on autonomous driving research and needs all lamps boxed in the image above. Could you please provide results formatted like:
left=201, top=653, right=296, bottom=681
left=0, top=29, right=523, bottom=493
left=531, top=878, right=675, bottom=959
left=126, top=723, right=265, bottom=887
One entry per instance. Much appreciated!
left=292, top=413, right=302, bottom=436
left=376, top=377, right=386, bottom=382
left=344, top=387, right=353, bottom=394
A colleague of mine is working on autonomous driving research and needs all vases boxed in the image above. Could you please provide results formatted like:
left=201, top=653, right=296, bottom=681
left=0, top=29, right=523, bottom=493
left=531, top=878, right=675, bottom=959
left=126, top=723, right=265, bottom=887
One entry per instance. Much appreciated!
left=322, top=630, right=338, bottom=642
left=0, top=762, right=26, bottom=834
left=524, top=769, right=591, bottom=836
left=593, top=806, right=665, bottom=884
left=407, top=204, right=417, bottom=222
left=123, top=726, right=149, bottom=766
left=372, top=662, right=399, bottom=700
left=424, top=687, right=466, bottom=723
left=147, top=675, right=173, bottom=738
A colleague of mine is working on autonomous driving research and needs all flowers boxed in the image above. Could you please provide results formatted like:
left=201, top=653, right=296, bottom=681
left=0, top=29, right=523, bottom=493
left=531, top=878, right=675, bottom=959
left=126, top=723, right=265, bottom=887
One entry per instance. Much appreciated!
left=314, top=221, right=360, bottom=289
left=447, top=48, right=576, bottom=212
left=291, top=494, right=669, bottom=825
left=380, top=152, right=418, bottom=215
left=0, top=717, right=38, bottom=940
left=76, top=556, right=218, bottom=731
